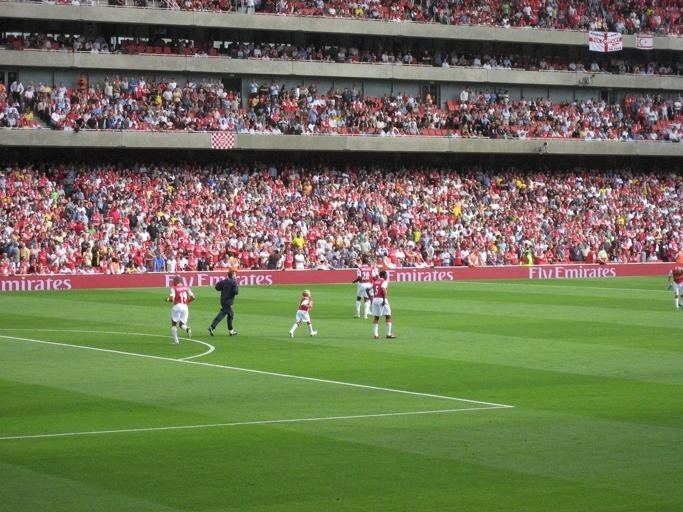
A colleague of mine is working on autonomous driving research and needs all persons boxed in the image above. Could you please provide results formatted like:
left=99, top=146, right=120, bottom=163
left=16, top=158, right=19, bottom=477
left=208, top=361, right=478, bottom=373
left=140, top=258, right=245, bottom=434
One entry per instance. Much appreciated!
left=1, top=148, right=682, bottom=276
left=1, top=77, right=682, bottom=143
left=349, top=255, right=373, bottom=319
left=163, top=277, right=195, bottom=344
left=364, top=271, right=394, bottom=338
left=2, top=1, right=681, bottom=75
left=666, top=253, right=682, bottom=308
left=207, top=270, right=238, bottom=336
left=286, top=289, right=318, bottom=338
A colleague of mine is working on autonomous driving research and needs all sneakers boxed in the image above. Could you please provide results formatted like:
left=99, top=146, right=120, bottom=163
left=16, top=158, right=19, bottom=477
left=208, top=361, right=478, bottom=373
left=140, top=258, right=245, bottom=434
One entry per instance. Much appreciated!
left=354, top=312, right=372, bottom=319
left=229, top=332, right=237, bottom=336
left=208, top=327, right=214, bottom=336
left=373, top=335, right=379, bottom=339
left=386, top=335, right=396, bottom=338
left=186, top=327, right=192, bottom=339
left=310, top=331, right=317, bottom=336
left=289, top=331, right=294, bottom=339
left=171, top=341, right=179, bottom=344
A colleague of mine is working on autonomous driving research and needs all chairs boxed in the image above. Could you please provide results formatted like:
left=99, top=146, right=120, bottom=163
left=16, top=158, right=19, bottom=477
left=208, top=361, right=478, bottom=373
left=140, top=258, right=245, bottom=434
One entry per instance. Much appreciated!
left=0, top=36, right=223, bottom=56
left=125, top=121, right=576, bottom=141
